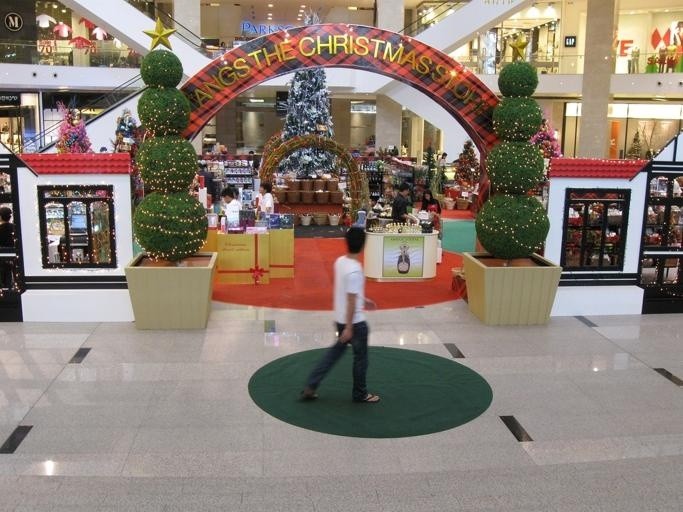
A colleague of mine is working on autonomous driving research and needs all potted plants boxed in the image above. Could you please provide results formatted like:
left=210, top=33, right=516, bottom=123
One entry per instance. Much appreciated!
left=125, top=50, right=218, bottom=328
left=463, top=61, right=564, bottom=325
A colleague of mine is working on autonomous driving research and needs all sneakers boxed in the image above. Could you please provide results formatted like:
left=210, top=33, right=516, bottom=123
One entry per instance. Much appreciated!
left=299, top=388, right=320, bottom=402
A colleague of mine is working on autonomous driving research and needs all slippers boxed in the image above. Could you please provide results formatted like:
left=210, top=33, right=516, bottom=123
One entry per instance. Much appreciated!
left=351, top=392, right=381, bottom=404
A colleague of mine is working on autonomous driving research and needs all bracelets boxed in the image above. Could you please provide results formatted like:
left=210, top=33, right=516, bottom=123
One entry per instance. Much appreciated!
left=344, top=325, right=353, bottom=328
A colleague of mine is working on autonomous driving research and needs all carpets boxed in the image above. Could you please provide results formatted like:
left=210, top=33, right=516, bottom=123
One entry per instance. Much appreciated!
left=249, top=345, right=493, bottom=438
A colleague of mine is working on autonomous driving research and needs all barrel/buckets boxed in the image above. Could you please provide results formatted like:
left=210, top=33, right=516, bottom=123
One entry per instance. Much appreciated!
left=274, top=178, right=344, bottom=227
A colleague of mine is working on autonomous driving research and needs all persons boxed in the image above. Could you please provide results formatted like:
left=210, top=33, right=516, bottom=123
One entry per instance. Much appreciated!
left=199, top=134, right=465, bottom=227
left=300, top=228, right=380, bottom=403
left=219, top=41, right=226, bottom=55
left=627, top=39, right=677, bottom=73
left=0, top=206, right=14, bottom=284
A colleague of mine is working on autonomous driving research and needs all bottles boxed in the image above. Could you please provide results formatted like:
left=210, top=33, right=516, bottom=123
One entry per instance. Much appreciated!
left=206, top=200, right=266, bottom=233
left=192, top=158, right=254, bottom=193
left=358, top=162, right=381, bottom=201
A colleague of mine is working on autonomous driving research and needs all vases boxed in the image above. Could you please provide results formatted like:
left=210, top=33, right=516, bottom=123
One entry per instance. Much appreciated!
left=298, top=213, right=341, bottom=227
left=274, top=177, right=343, bottom=205
